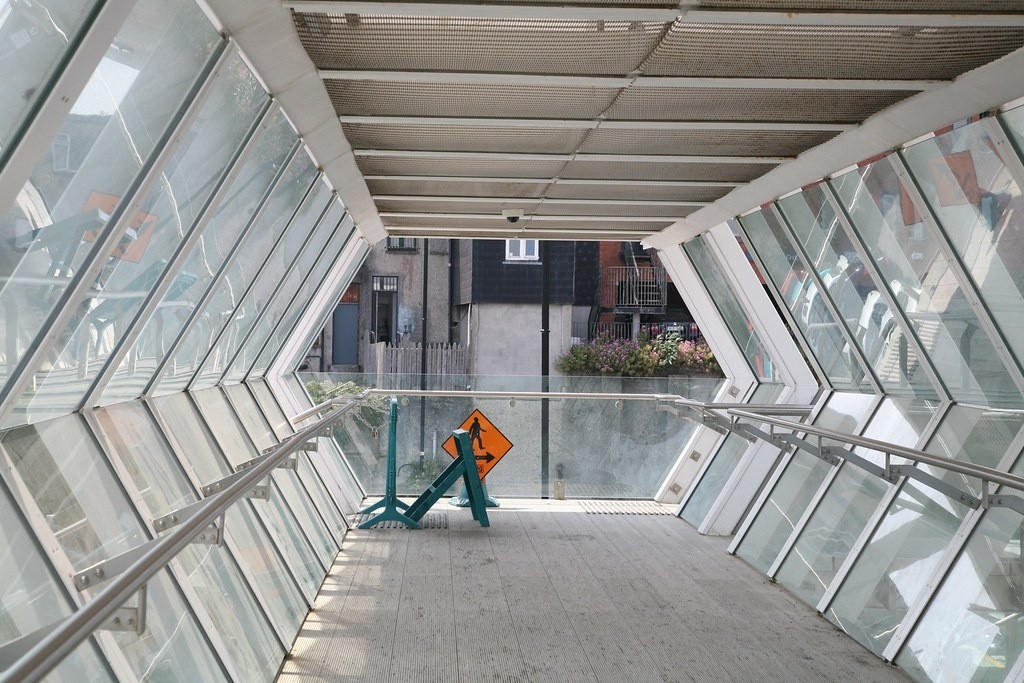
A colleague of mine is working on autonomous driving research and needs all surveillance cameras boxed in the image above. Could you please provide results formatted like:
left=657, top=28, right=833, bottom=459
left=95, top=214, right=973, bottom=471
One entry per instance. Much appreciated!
left=503, top=209, right=523, bottom=224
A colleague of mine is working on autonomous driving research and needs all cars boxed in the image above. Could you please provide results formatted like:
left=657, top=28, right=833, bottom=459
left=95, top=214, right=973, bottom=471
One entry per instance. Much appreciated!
left=642, top=305, right=698, bottom=343
